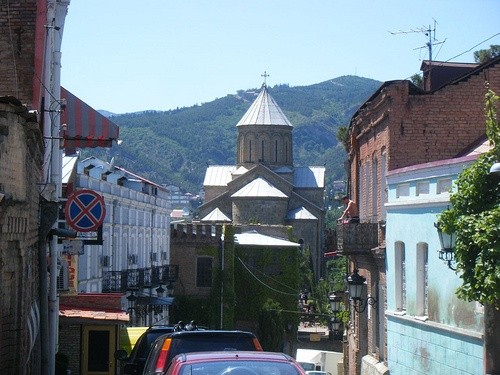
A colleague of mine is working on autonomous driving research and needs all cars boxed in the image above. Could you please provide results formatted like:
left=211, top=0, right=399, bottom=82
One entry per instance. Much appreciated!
left=161, top=351, right=307, bottom=375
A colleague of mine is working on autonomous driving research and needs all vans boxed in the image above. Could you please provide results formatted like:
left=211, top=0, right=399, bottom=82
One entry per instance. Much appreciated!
left=143, top=321, right=263, bottom=375
left=116, top=325, right=210, bottom=375
